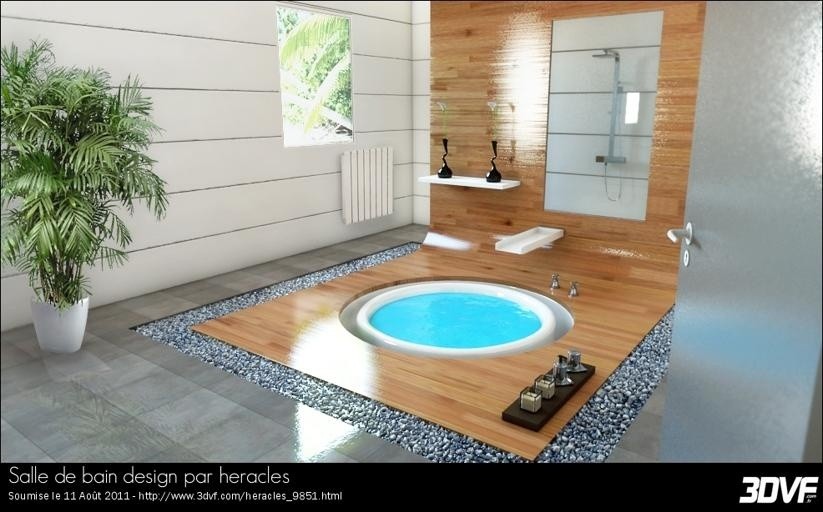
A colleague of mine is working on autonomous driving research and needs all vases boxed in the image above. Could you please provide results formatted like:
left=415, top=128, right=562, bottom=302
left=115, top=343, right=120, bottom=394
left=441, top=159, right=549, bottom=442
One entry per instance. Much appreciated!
left=485, top=141, right=501, bottom=183
left=437, top=139, right=452, bottom=178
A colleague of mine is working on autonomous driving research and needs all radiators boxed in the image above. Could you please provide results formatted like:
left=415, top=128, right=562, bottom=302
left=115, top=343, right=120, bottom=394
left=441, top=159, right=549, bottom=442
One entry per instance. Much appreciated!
left=339, top=145, right=394, bottom=225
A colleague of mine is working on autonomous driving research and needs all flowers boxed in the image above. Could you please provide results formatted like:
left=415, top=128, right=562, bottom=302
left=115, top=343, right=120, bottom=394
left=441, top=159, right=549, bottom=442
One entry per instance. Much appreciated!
left=437, top=100, right=453, bottom=139
left=486, top=101, right=498, bottom=141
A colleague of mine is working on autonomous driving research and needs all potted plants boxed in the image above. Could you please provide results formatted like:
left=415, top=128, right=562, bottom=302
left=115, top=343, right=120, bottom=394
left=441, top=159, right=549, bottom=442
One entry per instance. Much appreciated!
left=0, top=33, right=169, bottom=355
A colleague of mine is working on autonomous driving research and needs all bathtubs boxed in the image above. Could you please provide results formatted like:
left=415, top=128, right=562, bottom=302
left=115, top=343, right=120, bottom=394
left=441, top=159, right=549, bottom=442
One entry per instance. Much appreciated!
left=337, top=275, right=575, bottom=358
